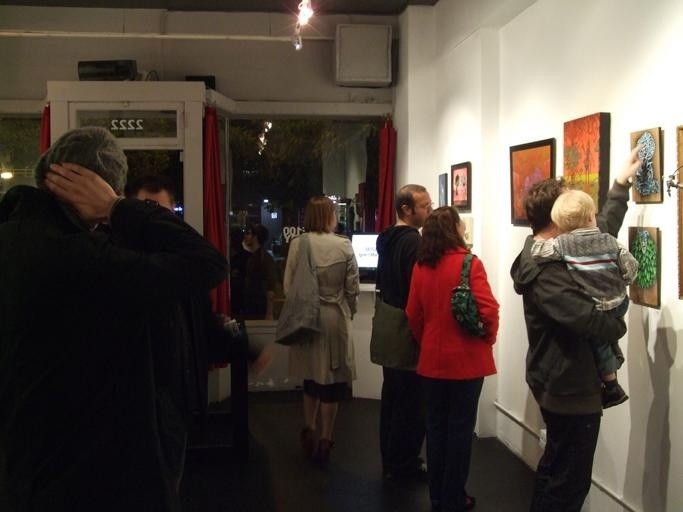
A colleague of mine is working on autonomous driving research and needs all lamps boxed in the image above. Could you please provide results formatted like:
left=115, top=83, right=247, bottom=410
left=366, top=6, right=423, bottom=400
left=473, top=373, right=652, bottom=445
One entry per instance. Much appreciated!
left=297, top=0, right=314, bottom=26
left=291, top=33, right=303, bottom=50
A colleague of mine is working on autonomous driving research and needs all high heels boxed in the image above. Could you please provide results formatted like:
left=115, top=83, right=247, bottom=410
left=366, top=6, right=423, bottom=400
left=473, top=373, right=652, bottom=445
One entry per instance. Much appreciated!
left=318, top=439, right=334, bottom=470
left=300, top=426, right=316, bottom=458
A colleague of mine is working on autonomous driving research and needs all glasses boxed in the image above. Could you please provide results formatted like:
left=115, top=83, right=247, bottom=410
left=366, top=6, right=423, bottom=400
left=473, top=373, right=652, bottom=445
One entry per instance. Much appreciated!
left=410, top=202, right=434, bottom=210
left=245, top=231, right=252, bottom=236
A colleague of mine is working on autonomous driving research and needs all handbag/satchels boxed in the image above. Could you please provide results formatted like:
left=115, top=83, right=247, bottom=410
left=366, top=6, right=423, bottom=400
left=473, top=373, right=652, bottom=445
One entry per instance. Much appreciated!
left=367, top=227, right=423, bottom=373
left=450, top=253, right=486, bottom=337
left=274, top=233, right=321, bottom=346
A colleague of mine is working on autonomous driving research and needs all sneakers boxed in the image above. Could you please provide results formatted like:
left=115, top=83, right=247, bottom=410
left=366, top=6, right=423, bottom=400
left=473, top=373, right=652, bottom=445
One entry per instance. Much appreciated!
left=601, top=384, right=629, bottom=410
left=463, top=493, right=475, bottom=512
left=408, top=461, right=429, bottom=480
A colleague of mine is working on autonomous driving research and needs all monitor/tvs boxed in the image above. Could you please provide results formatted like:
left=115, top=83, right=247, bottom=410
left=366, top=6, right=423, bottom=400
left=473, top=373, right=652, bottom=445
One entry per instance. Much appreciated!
left=352, top=233, right=382, bottom=271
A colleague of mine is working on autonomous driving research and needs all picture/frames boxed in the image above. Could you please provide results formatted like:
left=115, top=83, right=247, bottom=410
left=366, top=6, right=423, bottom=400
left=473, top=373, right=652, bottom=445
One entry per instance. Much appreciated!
left=628, top=226, right=661, bottom=308
left=450, top=162, right=471, bottom=209
left=563, top=113, right=610, bottom=213
left=630, top=127, right=662, bottom=204
left=438, top=173, right=447, bottom=207
left=510, top=138, right=556, bottom=224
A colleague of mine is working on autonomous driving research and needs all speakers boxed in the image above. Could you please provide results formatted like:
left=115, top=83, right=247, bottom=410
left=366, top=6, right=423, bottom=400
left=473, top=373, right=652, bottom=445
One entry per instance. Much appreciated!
left=334, top=23, right=392, bottom=87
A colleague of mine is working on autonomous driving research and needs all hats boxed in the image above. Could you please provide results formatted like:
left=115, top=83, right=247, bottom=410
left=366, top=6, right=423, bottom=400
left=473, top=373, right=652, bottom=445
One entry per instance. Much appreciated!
left=33, top=123, right=128, bottom=201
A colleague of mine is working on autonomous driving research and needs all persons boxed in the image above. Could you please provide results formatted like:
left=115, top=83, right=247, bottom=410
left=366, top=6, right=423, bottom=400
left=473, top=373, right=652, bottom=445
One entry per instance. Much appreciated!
left=405, top=206, right=499, bottom=511
left=530, top=189, right=640, bottom=409
left=282, top=196, right=360, bottom=473
left=369, top=184, right=434, bottom=487
left=0, top=126, right=227, bottom=512
left=134, top=170, right=178, bottom=212
left=510, top=142, right=645, bottom=512
left=230, top=221, right=278, bottom=320
